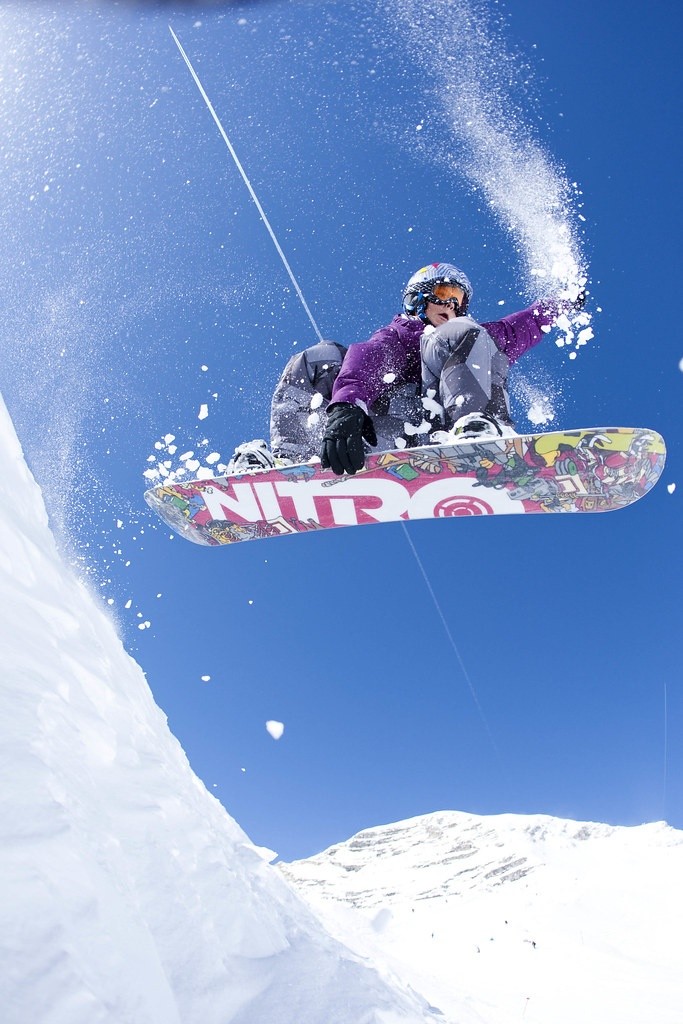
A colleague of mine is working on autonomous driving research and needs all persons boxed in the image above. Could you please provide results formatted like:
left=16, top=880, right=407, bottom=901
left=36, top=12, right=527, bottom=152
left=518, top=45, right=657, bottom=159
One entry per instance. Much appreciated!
left=228, top=262, right=587, bottom=479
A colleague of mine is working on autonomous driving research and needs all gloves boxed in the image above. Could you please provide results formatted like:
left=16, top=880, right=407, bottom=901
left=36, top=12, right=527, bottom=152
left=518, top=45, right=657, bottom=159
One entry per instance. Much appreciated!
left=574, top=287, right=586, bottom=312
left=322, top=402, right=378, bottom=477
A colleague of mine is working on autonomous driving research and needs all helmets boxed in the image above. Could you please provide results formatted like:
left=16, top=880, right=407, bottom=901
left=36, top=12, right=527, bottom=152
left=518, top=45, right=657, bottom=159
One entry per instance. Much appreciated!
left=402, top=263, right=473, bottom=315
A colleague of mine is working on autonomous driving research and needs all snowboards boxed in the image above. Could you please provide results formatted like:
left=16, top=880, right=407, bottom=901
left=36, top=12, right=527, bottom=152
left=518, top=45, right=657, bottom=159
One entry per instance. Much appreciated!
left=144, top=427, right=668, bottom=548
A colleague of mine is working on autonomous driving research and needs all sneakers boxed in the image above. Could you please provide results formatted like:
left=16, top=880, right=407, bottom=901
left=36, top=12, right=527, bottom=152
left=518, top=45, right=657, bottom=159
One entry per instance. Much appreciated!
left=234, top=440, right=294, bottom=473
left=454, top=411, right=517, bottom=441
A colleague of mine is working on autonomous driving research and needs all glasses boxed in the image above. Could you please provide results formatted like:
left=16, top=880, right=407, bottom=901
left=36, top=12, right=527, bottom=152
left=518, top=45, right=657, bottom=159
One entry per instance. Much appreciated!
left=423, top=282, right=465, bottom=312
